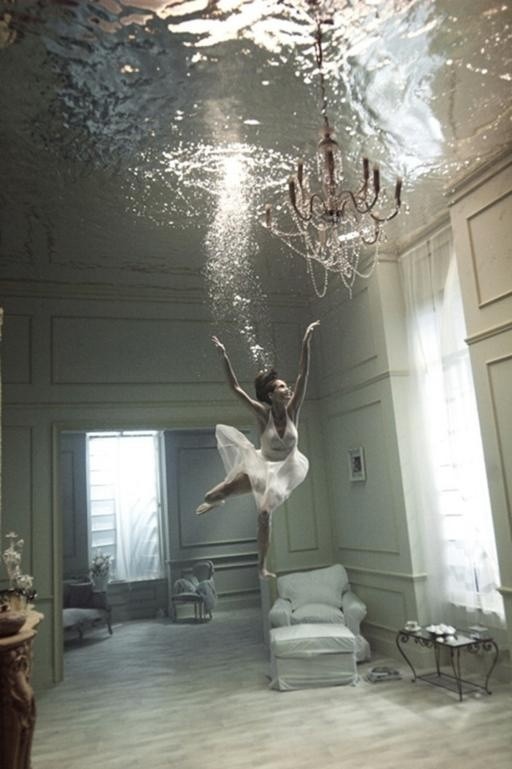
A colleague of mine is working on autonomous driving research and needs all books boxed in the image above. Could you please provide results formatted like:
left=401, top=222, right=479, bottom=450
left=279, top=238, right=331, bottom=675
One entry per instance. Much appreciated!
left=366, top=666, right=402, bottom=683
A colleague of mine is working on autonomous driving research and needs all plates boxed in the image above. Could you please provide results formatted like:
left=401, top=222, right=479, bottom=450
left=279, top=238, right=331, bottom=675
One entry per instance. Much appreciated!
left=405, top=627, right=421, bottom=631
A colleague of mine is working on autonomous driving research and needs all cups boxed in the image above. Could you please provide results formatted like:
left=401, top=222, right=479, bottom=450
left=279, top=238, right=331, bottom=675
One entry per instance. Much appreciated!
left=407, top=621, right=417, bottom=627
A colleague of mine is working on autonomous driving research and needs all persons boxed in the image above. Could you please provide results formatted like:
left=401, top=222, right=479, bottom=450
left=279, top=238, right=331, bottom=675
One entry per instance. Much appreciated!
left=194, top=319, right=324, bottom=582
